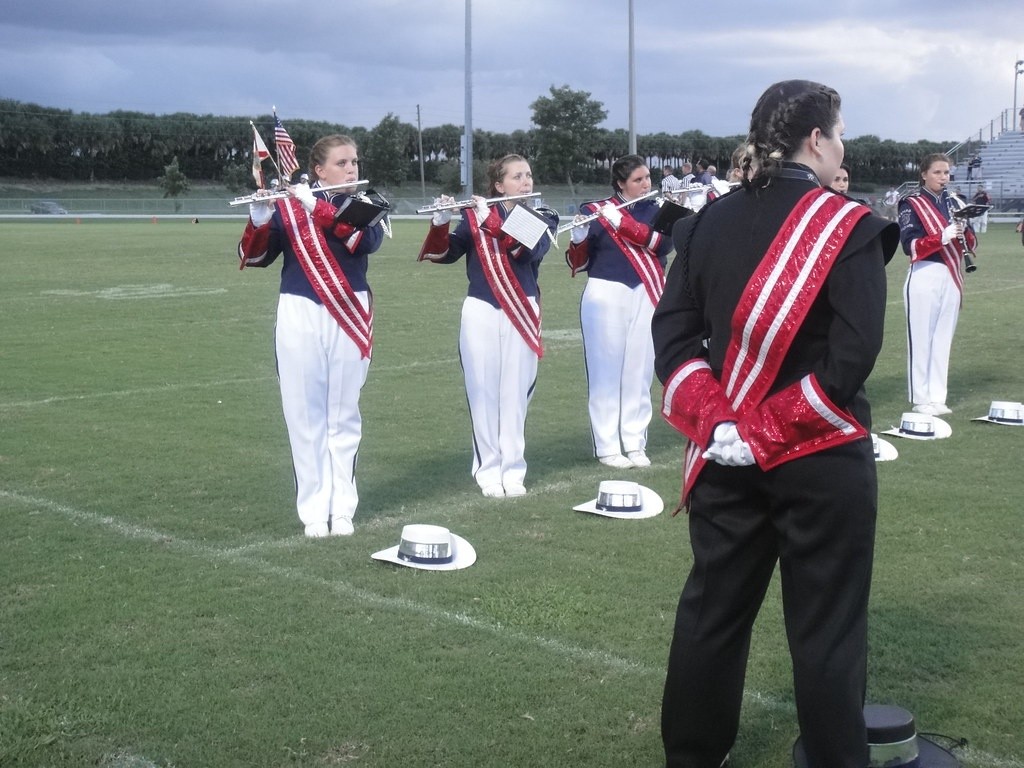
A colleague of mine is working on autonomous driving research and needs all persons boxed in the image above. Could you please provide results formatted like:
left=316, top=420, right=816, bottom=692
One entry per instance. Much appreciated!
left=566, top=155, right=676, bottom=469
left=897, top=152, right=978, bottom=415
left=650, top=81, right=900, bottom=767
left=415, top=153, right=561, bottom=496
left=868, top=185, right=900, bottom=222
left=236, top=135, right=394, bottom=538
left=948, top=153, right=989, bottom=233
left=661, top=141, right=848, bottom=215
left=270, top=174, right=309, bottom=193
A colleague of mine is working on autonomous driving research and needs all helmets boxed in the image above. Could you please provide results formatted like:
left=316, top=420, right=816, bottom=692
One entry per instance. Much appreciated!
left=301, top=174, right=308, bottom=180
left=283, top=174, right=291, bottom=182
left=270, top=179, right=279, bottom=185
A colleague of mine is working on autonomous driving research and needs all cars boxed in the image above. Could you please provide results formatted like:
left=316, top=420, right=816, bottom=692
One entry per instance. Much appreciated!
left=31, top=202, right=68, bottom=214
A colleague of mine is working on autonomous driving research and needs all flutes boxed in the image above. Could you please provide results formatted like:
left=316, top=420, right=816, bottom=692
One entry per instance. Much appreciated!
left=415, top=192, right=542, bottom=213
left=228, top=179, right=370, bottom=208
left=558, top=190, right=659, bottom=235
left=670, top=182, right=741, bottom=194
left=941, top=183, right=977, bottom=273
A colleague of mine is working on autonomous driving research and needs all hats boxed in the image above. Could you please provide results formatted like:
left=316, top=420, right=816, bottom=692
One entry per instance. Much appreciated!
left=793, top=703, right=969, bottom=768
left=371, top=522, right=477, bottom=570
left=871, top=432, right=900, bottom=462
left=969, top=400, right=1024, bottom=426
left=882, top=411, right=953, bottom=440
left=572, top=480, right=665, bottom=521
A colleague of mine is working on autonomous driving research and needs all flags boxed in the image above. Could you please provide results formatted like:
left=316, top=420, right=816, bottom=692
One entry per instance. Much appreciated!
left=274, top=112, right=299, bottom=176
left=252, top=124, right=270, bottom=191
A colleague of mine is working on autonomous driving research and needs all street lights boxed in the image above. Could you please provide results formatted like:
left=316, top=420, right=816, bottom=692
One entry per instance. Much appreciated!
left=1013, top=60, right=1024, bottom=131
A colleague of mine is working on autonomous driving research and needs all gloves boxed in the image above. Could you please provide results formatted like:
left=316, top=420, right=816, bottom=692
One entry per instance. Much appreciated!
left=702, top=422, right=757, bottom=467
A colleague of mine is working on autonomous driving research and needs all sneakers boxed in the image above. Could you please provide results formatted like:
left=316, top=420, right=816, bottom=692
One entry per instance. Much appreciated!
left=912, top=404, right=939, bottom=415
left=484, top=483, right=508, bottom=499
left=503, top=482, right=527, bottom=499
left=933, top=403, right=952, bottom=416
left=304, top=522, right=330, bottom=538
left=630, top=448, right=651, bottom=467
left=600, top=454, right=633, bottom=469
left=331, top=514, right=353, bottom=537
